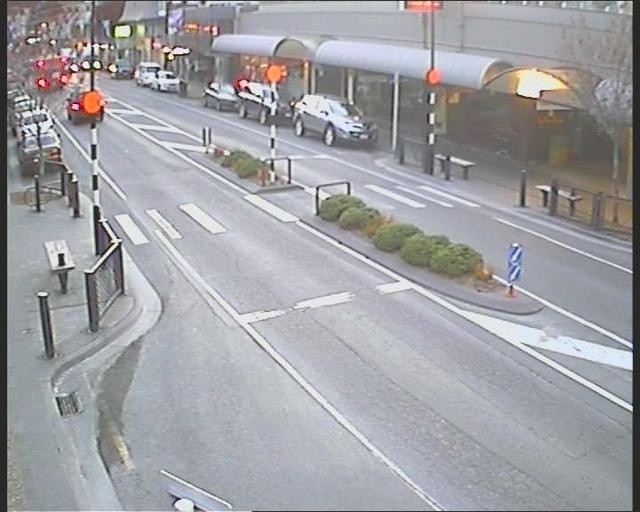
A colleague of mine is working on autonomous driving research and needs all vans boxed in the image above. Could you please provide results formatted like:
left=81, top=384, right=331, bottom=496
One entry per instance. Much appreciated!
left=66, top=89, right=105, bottom=125
left=34, top=56, right=72, bottom=88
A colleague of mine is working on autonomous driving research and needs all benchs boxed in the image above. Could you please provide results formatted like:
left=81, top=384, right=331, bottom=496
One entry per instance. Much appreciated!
left=44, top=238, right=77, bottom=294
left=434, top=153, right=475, bottom=179
left=535, top=184, right=582, bottom=214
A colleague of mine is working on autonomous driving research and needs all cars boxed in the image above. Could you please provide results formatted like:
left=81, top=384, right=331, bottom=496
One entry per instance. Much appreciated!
left=78, top=54, right=182, bottom=94
left=201, top=78, right=380, bottom=147
left=7, top=69, right=62, bottom=178
